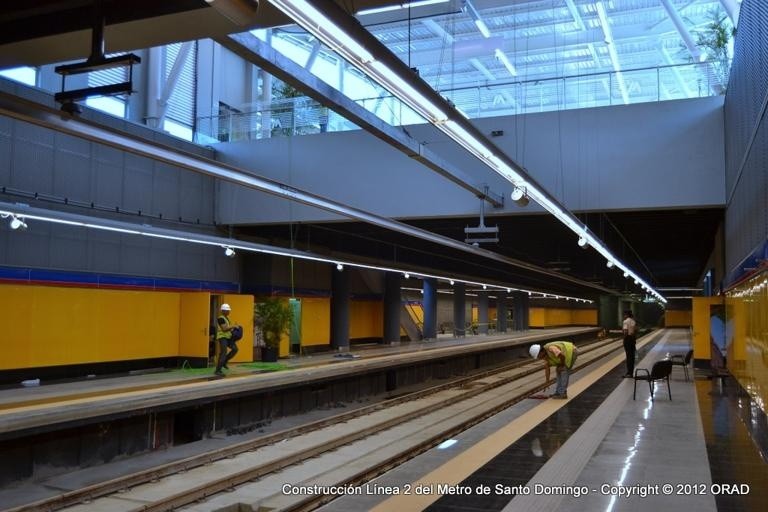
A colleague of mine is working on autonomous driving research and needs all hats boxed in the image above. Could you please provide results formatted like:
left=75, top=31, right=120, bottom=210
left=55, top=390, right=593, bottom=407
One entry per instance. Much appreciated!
left=623, top=309, right=632, bottom=315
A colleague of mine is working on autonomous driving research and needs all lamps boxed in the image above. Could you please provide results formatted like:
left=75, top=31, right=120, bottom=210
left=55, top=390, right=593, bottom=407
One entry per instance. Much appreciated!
left=510, top=186, right=529, bottom=207
left=577, top=236, right=588, bottom=249
left=225, top=246, right=235, bottom=258
left=11, top=214, right=27, bottom=232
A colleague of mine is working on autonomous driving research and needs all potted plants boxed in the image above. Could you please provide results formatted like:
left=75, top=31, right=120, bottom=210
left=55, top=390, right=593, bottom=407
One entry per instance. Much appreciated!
left=251, top=293, right=296, bottom=362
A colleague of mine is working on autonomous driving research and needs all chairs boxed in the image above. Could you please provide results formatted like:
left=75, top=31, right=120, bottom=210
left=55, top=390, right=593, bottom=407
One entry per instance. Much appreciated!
left=632, top=348, right=694, bottom=402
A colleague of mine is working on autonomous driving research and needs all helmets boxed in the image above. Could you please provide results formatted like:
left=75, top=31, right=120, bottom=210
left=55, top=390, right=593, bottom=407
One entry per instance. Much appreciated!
left=221, top=304, right=231, bottom=310
left=529, top=344, right=541, bottom=360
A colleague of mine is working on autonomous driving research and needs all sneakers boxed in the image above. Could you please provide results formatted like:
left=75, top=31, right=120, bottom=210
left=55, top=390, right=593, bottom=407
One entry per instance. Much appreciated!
left=549, top=391, right=567, bottom=399
left=214, top=362, right=229, bottom=377
left=622, top=374, right=634, bottom=378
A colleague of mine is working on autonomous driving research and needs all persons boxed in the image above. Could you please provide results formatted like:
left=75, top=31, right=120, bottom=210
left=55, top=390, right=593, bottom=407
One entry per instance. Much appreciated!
left=620, top=309, right=637, bottom=378
left=212, top=303, right=240, bottom=375
left=528, top=341, right=579, bottom=399
